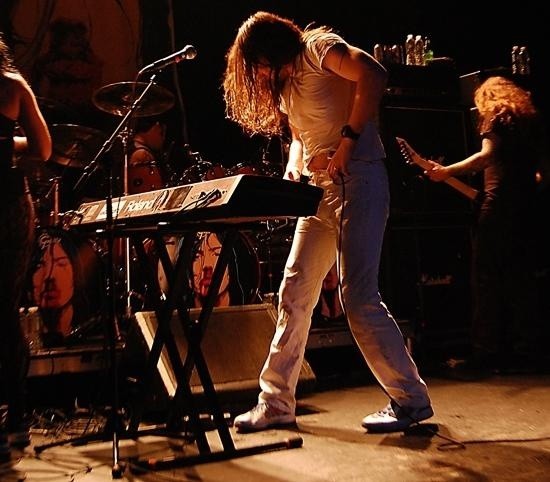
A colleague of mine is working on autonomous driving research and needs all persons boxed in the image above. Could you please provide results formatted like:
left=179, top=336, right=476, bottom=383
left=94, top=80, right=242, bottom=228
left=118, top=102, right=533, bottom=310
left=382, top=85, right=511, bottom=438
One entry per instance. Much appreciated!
left=223, top=10, right=434, bottom=432
left=188, top=231, right=238, bottom=310
left=0, top=36, right=53, bottom=469
left=320, top=263, right=343, bottom=319
left=98, top=112, right=178, bottom=294
left=22, top=229, right=90, bottom=344
left=424, top=73, right=550, bottom=378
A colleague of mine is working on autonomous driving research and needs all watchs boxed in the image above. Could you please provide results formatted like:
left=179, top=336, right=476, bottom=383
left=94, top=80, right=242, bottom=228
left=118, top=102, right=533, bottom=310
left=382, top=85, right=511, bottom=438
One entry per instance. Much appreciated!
left=341, top=125, right=364, bottom=143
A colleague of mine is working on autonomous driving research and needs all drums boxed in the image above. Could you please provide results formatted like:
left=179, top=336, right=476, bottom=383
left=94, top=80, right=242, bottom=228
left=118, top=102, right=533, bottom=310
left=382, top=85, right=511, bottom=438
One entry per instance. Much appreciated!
left=130, top=231, right=261, bottom=307
left=261, top=220, right=346, bottom=323
left=19, top=227, right=110, bottom=336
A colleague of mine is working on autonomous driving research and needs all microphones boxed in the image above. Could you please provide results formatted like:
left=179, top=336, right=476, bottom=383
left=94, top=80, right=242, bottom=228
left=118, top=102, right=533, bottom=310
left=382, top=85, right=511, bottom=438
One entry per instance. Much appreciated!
left=143, top=44, right=197, bottom=73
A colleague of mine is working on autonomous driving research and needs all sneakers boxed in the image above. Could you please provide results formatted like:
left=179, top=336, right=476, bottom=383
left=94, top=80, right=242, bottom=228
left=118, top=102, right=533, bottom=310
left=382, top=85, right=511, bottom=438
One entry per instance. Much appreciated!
left=361, top=403, right=436, bottom=429
left=235, top=404, right=297, bottom=431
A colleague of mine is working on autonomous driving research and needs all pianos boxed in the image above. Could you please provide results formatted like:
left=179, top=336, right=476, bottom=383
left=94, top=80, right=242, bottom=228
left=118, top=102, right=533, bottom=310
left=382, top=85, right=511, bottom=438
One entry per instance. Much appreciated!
left=68, top=174, right=324, bottom=238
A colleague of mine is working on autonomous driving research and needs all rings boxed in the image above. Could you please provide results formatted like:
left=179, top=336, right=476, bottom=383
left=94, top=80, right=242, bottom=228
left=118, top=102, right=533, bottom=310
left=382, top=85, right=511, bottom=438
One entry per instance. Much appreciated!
left=333, top=167, right=339, bottom=172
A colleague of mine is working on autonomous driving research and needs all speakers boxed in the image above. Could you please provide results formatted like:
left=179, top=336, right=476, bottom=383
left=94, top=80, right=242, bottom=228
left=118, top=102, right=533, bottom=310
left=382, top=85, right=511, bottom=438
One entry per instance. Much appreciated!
left=117, top=302, right=317, bottom=425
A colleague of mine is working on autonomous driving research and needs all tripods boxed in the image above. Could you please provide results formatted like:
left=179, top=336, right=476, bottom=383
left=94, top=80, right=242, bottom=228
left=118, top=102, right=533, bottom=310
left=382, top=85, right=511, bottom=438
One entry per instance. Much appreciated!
left=33, top=73, right=195, bottom=479
left=64, top=110, right=162, bottom=347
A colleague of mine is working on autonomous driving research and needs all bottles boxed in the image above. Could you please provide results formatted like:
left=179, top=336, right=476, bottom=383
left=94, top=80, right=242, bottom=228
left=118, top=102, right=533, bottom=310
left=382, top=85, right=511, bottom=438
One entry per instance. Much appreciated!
left=19, top=307, right=26, bottom=341
left=405, top=33, right=416, bottom=66
left=518, top=46, right=531, bottom=75
left=423, top=39, right=434, bottom=65
left=24, top=306, right=44, bottom=352
left=414, top=34, right=426, bottom=66
left=510, top=45, right=521, bottom=75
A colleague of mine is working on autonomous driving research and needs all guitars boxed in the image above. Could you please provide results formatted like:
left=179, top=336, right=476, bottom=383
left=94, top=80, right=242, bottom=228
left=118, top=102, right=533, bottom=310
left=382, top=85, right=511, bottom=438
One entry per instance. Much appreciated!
left=397, top=138, right=488, bottom=212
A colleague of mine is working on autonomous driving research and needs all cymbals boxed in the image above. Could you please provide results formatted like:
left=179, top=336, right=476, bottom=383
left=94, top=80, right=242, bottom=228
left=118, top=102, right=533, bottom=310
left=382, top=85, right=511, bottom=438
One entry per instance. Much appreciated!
left=47, top=124, right=111, bottom=166
left=93, top=82, right=175, bottom=117
left=14, top=157, right=64, bottom=188
